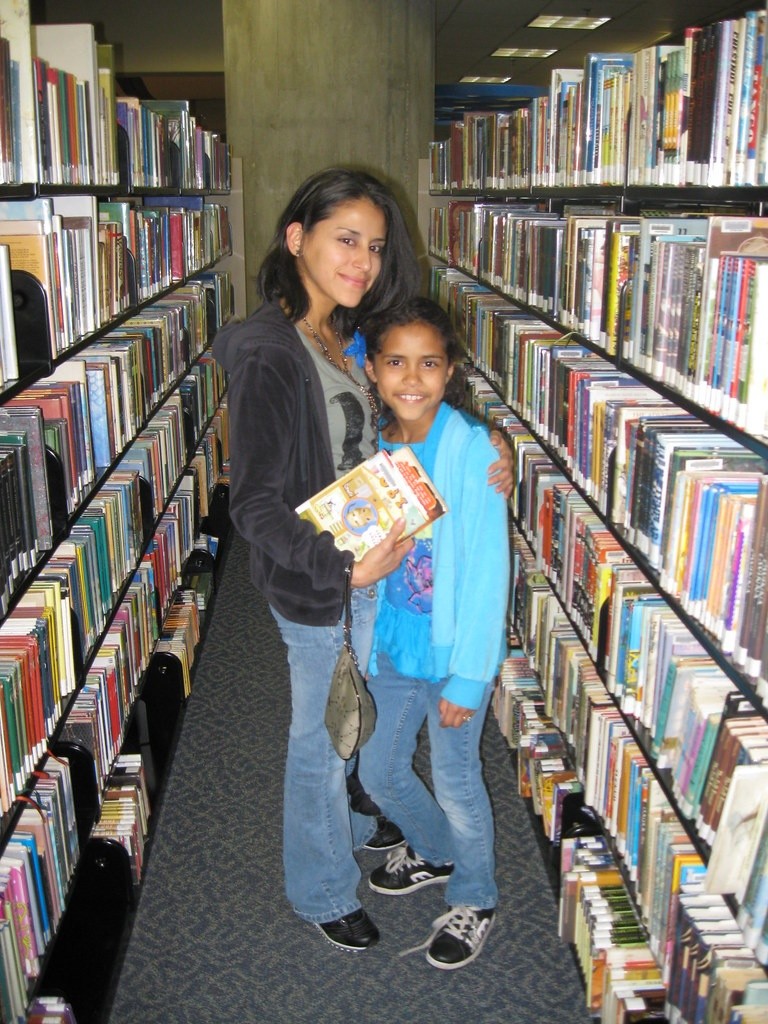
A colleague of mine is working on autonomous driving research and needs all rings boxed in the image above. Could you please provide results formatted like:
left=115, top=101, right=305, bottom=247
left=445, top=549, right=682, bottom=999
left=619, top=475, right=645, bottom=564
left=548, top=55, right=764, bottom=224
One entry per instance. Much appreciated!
left=462, top=711, right=472, bottom=722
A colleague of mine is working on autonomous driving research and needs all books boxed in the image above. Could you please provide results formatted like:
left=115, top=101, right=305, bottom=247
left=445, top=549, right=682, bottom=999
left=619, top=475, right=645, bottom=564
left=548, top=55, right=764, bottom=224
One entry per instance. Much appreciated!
left=291, top=444, right=448, bottom=563
left=0, top=33, right=230, bottom=1023
left=425, top=10, right=768, bottom=1023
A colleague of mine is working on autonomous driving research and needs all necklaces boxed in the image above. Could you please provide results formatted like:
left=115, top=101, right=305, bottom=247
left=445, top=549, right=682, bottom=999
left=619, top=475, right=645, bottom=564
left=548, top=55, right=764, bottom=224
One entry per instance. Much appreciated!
left=296, top=310, right=382, bottom=457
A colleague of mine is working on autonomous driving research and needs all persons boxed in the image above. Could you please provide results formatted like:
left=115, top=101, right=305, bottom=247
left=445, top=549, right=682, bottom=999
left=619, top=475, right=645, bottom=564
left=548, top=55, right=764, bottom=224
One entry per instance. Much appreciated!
left=355, top=295, right=512, bottom=970
left=211, top=165, right=516, bottom=958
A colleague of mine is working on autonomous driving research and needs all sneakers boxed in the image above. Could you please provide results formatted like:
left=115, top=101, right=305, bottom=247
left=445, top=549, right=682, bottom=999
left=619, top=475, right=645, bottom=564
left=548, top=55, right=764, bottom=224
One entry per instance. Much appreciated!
left=369, top=845, right=455, bottom=895
left=398, top=905, right=496, bottom=969
left=364, top=816, right=405, bottom=850
left=313, top=907, right=379, bottom=952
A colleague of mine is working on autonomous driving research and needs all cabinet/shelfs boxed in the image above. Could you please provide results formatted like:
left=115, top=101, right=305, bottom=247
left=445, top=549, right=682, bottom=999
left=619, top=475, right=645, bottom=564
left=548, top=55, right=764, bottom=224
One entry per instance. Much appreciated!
left=0, top=154, right=246, bottom=1024
left=416, top=156, right=767, bottom=1023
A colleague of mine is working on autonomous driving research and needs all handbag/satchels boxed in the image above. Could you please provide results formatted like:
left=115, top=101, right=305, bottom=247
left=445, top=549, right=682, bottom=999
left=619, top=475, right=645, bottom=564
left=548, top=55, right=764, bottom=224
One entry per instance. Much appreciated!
left=325, top=560, right=376, bottom=760
